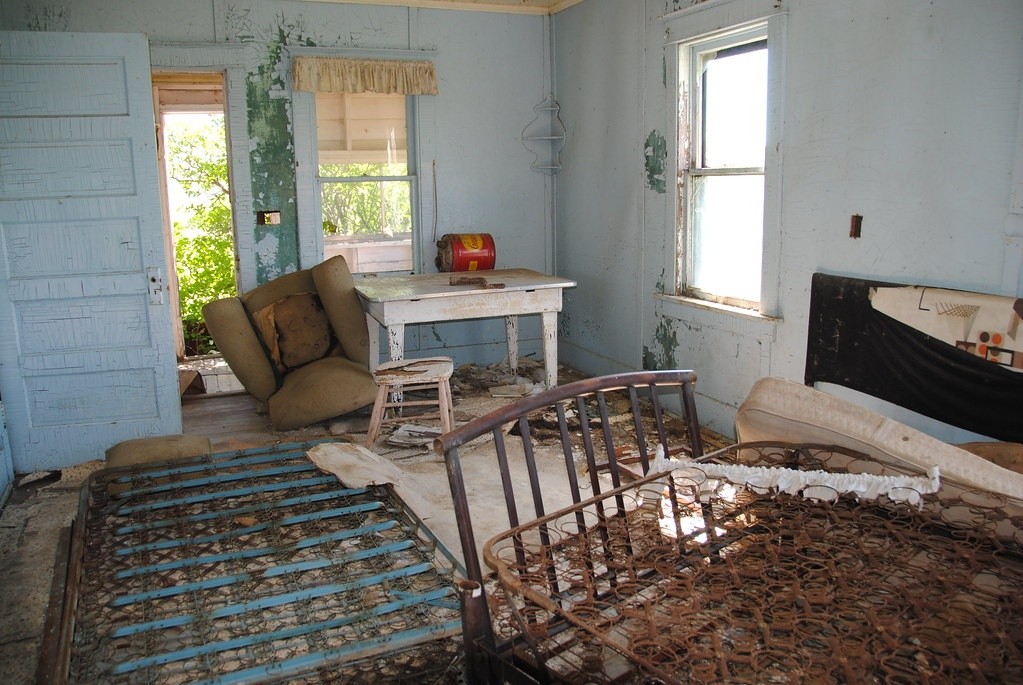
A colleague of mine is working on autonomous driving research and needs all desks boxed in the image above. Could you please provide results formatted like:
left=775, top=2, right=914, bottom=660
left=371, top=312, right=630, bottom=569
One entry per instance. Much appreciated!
left=354, top=267, right=578, bottom=391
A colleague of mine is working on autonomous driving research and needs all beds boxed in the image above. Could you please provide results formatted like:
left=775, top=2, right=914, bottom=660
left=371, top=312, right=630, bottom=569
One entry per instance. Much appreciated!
left=434, top=367, right=1022, bottom=685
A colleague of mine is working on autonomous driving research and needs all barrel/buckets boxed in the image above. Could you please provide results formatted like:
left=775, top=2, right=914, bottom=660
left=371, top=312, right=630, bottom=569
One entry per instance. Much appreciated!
left=434, top=232, right=496, bottom=272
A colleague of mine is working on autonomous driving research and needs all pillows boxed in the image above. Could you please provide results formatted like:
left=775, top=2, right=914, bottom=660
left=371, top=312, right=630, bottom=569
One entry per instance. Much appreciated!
left=105, top=434, right=213, bottom=494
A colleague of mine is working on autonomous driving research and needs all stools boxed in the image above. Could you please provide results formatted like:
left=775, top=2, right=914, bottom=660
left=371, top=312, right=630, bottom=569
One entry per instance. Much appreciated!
left=364, top=355, right=455, bottom=449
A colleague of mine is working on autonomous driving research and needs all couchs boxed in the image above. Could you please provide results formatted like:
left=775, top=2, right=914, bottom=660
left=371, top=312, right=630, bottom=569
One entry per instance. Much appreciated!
left=201, top=254, right=381, bottom=431
